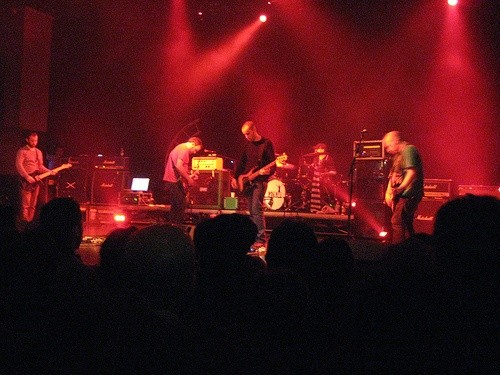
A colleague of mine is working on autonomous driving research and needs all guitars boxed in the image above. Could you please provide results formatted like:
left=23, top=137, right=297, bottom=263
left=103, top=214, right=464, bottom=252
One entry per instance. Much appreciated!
left=238, top=154, right=288, bottom=193
left=178, top=169, right=199, bottom=195
left=14, top=163, right=72, bottom=193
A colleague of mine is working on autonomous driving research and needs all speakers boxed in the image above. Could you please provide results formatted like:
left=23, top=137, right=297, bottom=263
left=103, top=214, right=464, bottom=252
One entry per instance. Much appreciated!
left=56, top=169, right=91, bottom=204
left=412, top=197, right=448, bottom=235
left=189, top=170, right=230, bottom=210
left=91, top=171, right=130, bottom=205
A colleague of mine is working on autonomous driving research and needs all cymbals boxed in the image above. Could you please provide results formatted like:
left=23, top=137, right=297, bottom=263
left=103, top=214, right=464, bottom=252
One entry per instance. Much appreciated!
left=303, top=151, right=330, bottom=157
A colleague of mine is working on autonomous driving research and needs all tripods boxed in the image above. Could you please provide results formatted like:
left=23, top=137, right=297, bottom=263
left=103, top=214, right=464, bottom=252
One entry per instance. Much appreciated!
left=315, top=134, right=383, bottom=245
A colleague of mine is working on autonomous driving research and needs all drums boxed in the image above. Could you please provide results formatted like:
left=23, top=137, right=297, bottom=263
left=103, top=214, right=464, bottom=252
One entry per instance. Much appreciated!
left=263, top=179, right=287, bottom=210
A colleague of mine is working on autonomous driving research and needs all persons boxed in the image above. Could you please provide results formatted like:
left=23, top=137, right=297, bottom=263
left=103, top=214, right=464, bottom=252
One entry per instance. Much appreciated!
left=45, top=143, right=70, bottom=195
left=382, top=131, right=424, bottom=245
left=15, top=131, right=57, bottom=229
left=162, top=137, right=203, bottom=226
left=305, top=143, right=337, bottom=176
left=231, top=121, right=277, bottom=246
left=0, top=173, right=500, bottom=375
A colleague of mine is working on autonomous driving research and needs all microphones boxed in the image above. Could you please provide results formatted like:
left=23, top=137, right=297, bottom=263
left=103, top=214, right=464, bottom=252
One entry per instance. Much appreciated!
left=361, top=130, right=367, bottom=134
